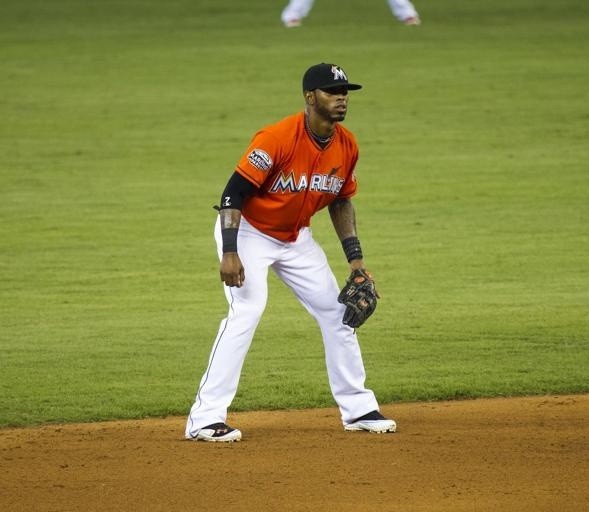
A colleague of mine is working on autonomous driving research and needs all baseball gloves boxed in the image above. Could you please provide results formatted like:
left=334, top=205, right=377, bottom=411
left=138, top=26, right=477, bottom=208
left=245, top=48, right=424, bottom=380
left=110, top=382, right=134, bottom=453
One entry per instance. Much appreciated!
left=338, top=269, right=380, bottom=334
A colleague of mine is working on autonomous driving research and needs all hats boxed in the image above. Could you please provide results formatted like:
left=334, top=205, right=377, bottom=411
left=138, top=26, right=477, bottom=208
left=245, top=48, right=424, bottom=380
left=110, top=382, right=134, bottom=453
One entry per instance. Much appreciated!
left=300, top=59, right=362, bottom=93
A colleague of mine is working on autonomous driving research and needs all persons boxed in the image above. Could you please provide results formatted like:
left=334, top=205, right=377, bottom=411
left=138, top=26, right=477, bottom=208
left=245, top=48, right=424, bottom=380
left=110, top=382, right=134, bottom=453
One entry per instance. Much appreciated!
left=176, top=62, right=399, bottom=443
left=278, top=0, right=423, bottom=30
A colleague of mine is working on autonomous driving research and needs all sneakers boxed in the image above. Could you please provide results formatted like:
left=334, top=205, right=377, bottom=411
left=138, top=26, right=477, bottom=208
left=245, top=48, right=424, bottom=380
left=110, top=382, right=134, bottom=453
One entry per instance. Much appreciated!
left=342, top=408, right=398, bottom=434
left=185, top=419, right=243, bottom=442
left=283, top=13, right=420, bottom=29
